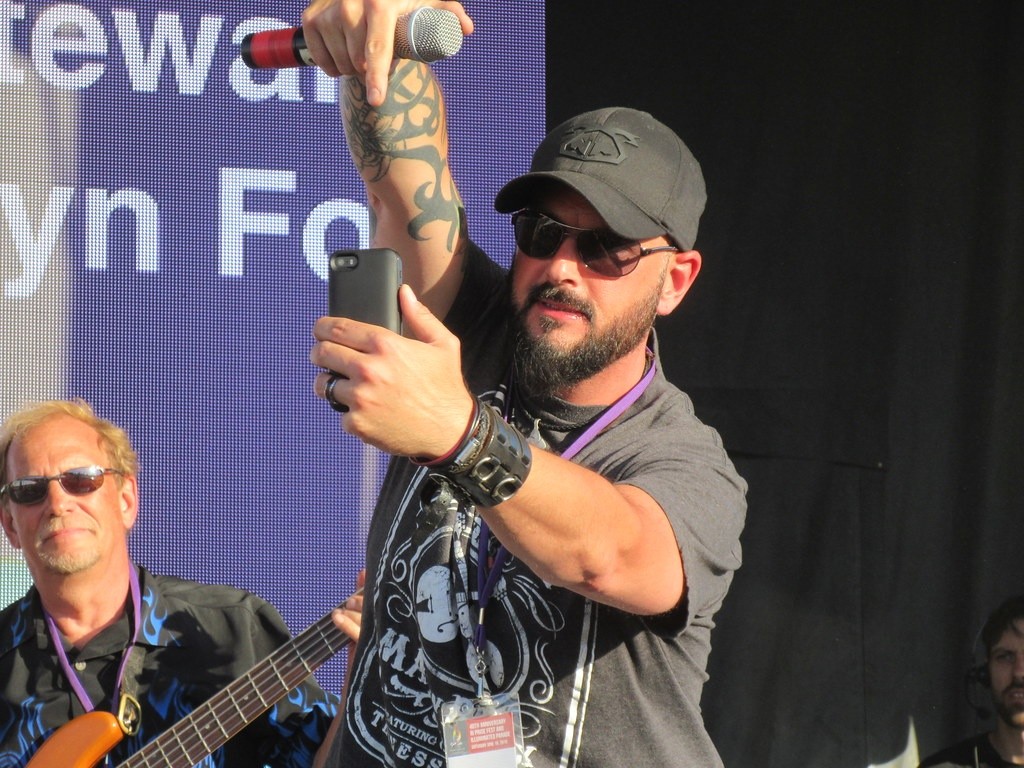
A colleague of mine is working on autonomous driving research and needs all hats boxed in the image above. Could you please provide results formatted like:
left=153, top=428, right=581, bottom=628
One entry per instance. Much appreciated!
left=495, top=107, right=707, bottom=251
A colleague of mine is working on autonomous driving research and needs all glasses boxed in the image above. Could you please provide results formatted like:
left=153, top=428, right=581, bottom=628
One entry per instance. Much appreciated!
left=512, top=210, right=678, bottom=277
left=1, top=466, right=123, bottom=505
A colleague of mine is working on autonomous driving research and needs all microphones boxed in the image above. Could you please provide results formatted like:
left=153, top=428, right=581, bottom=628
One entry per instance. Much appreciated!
left=241, top=7, right=464, bottom=69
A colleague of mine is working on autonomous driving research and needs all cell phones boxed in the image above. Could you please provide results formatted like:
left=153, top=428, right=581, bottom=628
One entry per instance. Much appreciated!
left=326, top=248, right=405, bottom=413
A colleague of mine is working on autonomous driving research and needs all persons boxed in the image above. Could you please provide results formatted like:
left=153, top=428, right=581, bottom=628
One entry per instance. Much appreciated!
left=918, top=595, right=1024, bottom=768
left=0, top=397, right=365, bottom=768
left=299, top=1, right=748, bottom=768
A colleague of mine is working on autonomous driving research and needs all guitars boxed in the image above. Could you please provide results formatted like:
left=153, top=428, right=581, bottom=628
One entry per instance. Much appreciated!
left=23, top=586, right=364, bottom=768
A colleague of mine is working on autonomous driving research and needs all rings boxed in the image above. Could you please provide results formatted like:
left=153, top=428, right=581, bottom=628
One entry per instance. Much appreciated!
left=323, top=376, right=349, bottom=413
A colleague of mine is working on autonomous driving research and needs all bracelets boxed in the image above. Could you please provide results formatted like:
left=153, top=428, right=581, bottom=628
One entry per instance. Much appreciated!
left=410, top=391, right=532, bottom=510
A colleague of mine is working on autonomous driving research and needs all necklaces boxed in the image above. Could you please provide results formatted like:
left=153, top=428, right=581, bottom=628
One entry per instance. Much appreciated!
left=43, top=558, right=141, bottom=768
